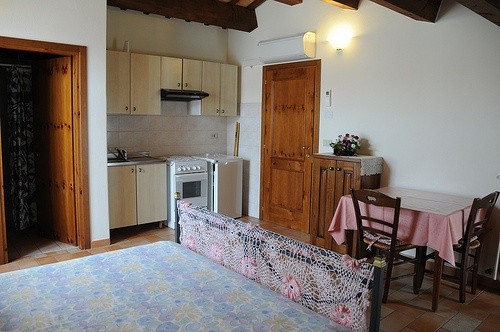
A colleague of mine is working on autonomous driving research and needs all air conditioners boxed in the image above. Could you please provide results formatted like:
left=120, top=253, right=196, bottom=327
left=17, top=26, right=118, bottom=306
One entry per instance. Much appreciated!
left=256, top=31, right=317, bottom=63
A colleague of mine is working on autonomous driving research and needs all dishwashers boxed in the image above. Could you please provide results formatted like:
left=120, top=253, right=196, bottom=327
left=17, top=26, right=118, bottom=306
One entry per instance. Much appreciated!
left=191, top=153, right=242, bottom=218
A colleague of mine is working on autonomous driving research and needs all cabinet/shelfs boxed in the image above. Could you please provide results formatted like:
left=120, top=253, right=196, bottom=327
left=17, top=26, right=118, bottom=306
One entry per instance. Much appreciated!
left=108, top=162, right=167, bottom=229
left=188, top=61, right=239, bottom=116
left=106, top=50, right=162, bottom=115
left=311, top=155, right=382, bottom=255
left=161, top=55, right=202, bottom=91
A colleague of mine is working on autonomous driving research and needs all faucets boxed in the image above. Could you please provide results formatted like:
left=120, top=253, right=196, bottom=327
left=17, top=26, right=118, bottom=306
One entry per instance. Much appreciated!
left=110, top=147, right=128, bottom=160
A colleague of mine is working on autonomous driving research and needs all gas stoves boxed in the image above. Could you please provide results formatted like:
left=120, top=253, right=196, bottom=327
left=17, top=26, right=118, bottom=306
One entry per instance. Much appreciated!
left=160, top=156, right=207, bottom=163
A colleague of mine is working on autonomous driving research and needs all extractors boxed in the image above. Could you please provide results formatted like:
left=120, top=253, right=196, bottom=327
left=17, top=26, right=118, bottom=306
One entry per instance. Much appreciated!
left=161, top=89, right=209, bottom=102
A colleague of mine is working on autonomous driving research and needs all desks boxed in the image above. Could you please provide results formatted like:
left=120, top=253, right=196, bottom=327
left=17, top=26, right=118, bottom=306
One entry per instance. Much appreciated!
left=342, top=186, right=481, bottom=312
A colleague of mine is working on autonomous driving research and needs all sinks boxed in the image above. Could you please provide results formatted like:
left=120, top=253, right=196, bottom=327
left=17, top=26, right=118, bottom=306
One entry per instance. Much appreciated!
left=107, top=157, right=131, bottom=166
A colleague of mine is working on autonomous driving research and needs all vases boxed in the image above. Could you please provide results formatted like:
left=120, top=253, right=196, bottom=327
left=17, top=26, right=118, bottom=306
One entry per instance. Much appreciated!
left=333, top=148, right=355, bottom=156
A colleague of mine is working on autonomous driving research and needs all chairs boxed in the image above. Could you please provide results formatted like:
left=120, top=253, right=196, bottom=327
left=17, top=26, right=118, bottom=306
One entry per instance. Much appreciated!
left=348, top=188, right=422, bottom=303
left=420, top=189, right=500, bottom=304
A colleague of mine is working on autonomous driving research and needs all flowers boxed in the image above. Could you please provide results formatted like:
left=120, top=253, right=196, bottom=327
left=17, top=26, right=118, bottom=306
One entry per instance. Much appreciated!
left=329, top=134, right=361, bottom=152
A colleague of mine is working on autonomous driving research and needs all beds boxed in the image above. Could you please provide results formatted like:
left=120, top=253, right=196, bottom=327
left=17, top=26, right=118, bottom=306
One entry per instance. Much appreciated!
left=0, top=192, right=387, bottom=332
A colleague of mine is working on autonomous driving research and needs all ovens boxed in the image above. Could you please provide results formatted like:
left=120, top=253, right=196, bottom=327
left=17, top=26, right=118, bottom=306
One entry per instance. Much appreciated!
left=165, top=166, right=208, bottom=229
